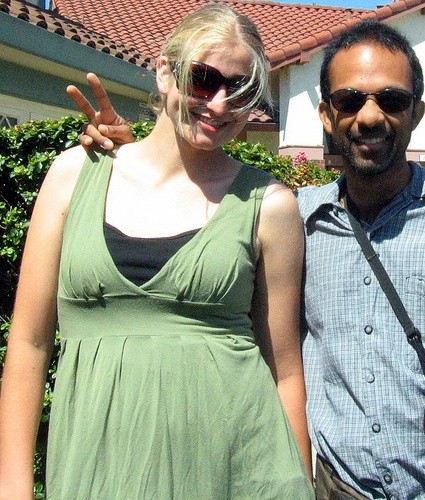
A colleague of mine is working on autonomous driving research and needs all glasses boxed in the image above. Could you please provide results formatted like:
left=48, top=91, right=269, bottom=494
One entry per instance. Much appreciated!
left=169, top=61, right=260, bottom=107
left=326, top=88, right=416, bottom=114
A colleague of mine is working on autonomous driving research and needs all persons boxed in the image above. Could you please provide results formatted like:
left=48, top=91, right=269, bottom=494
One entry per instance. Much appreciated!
left=0, top=3, right=316, bottom=500
left=65, top=17, right=425, bottom=500
